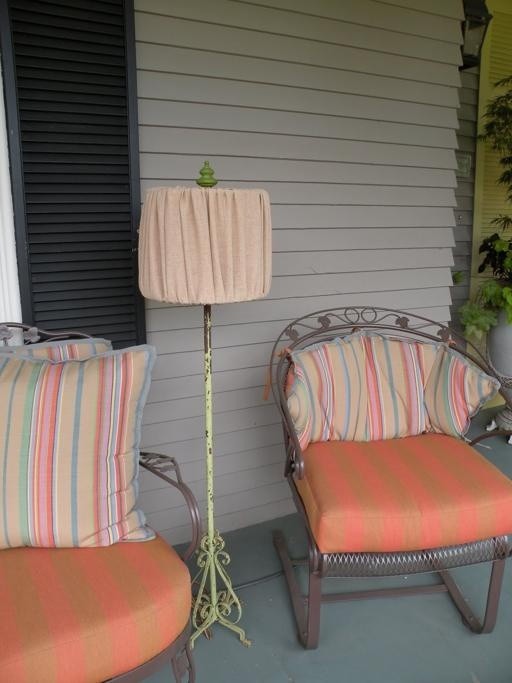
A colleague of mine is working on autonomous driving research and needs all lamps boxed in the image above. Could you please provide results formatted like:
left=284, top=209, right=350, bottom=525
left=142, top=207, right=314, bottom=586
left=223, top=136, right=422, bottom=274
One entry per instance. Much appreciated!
left=135, top=159, right=273, bottom=651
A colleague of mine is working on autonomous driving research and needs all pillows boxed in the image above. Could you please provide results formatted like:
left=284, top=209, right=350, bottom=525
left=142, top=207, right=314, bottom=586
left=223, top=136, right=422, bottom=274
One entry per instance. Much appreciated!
left=5, top=336, right=115, bottom=363
left=282, top=332, right=502, bottom=459
left=0, top=343, right=158, bottom=551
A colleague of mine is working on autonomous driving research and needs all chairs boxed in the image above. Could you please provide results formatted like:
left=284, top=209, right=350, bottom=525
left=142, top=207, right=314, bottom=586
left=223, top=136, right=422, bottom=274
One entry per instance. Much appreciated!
left=266, top=304, right=510, bottom=649
left=0, top=319, right=204, bottom=683
